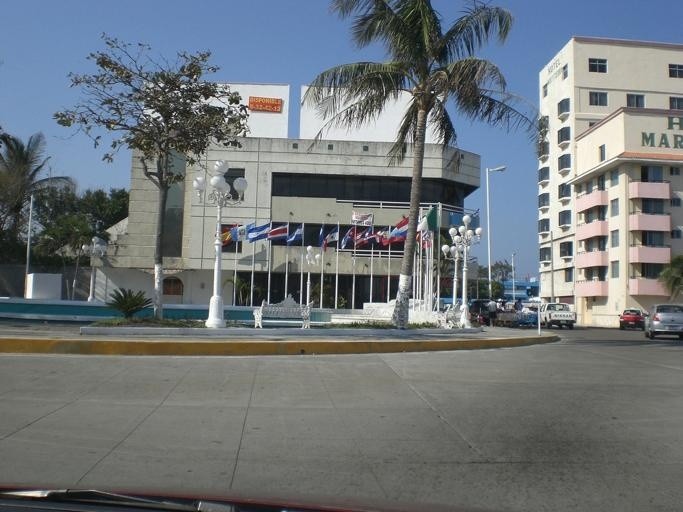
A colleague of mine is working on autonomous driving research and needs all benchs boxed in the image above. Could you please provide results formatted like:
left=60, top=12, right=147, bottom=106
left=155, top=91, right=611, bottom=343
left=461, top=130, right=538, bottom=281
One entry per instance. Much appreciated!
left=251, top=292, right=315, bottom=329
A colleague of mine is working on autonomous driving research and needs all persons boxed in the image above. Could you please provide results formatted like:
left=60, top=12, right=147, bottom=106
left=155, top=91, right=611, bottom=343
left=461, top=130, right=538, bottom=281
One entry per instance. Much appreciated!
left=470, top=295, right=522, bottom=328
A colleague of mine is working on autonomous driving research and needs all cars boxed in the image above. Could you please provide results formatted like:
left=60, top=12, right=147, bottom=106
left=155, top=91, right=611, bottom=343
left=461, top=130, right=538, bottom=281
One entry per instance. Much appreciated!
left=644, top=303, right=682, bottom=339
left=617, top=308, right=648, bottom=331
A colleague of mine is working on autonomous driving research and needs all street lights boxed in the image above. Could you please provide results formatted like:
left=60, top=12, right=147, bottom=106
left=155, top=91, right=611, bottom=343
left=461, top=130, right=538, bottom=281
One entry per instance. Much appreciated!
left=449, top=216, right=482, bottom=328
left=486, top=166, right=507, bottom=299
left=510, top=253, right=516, bottom=300
left=81, top=236, right=102, bottom=303
left=193, top=160, right=246, bottom=329
left=441, top=243, right=470, bottom=308
left=301, top=246, right=321, bottom=310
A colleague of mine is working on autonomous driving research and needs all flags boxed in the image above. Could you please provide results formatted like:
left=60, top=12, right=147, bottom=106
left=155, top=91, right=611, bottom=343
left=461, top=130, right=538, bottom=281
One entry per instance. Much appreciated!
left=215, top=222, right=302, bottom=248
left=317, top=216, right=433, bottom=253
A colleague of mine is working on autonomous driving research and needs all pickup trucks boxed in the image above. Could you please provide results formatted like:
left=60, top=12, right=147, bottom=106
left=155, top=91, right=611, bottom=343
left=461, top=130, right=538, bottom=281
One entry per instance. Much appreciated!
left=539, top=303, right=576, bottom=329
